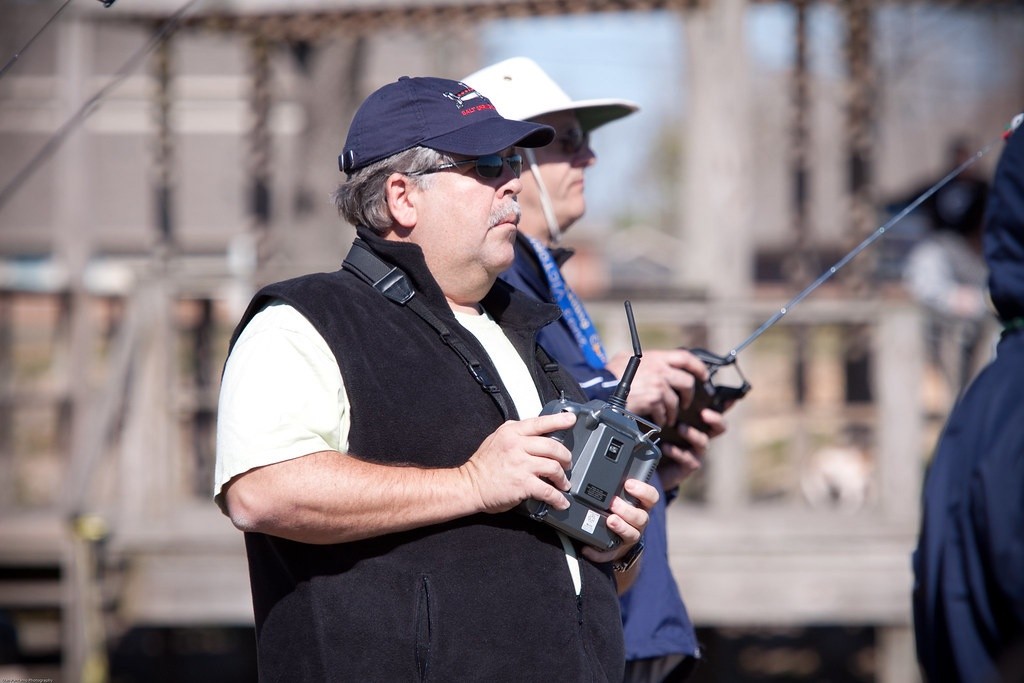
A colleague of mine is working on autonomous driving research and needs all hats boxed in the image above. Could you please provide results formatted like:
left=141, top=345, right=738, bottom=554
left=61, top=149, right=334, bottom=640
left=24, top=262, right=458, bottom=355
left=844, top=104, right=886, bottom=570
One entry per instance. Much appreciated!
left=458, top=57, right=639, bottom=130
left=338, top=76, right=557, bottom=174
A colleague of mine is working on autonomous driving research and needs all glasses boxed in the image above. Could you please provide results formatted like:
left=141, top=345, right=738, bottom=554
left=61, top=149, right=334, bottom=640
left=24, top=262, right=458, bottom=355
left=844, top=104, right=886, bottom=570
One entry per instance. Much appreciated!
left=556, top=127, right=588, bottom=153
left=385, top=153, right=523, bottom=202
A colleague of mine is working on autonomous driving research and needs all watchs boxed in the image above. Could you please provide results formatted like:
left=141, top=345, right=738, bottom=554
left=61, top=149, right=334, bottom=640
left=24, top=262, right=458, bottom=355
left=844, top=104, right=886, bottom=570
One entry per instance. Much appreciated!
left=611, top=542, right=645, bottom=574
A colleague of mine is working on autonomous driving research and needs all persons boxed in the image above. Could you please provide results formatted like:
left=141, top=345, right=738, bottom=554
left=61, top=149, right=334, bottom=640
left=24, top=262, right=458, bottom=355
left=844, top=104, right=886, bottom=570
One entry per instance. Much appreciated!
left=913, top=113, right=1024, bottom=683
left=213, top=76, right=660, bottom=683
left=459, top=56, right=729, bottom=682
